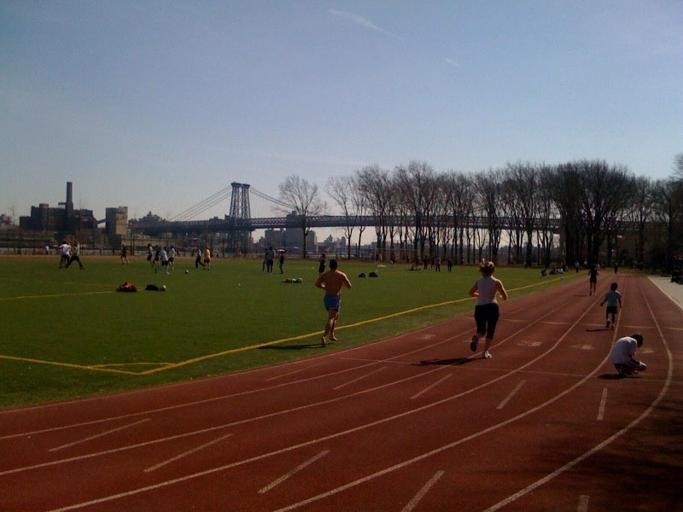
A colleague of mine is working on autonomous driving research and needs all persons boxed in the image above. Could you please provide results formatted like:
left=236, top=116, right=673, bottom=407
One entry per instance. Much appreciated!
left=609, top=333, right=647, bottom=379
left=317, top=252, right=326, bottom=277
left=540, top=257, right=640, bottom=276
left=56, top=239, right=286, bottom=278
left=314, top=258, right=351, bottom=346
left=376, top=251, right=453, bottom=273
left=587, top=264, right=598, bottom=296
left=468, top=260, right=507, bottom=360
left=600, top=282, right=623, bottom=330
